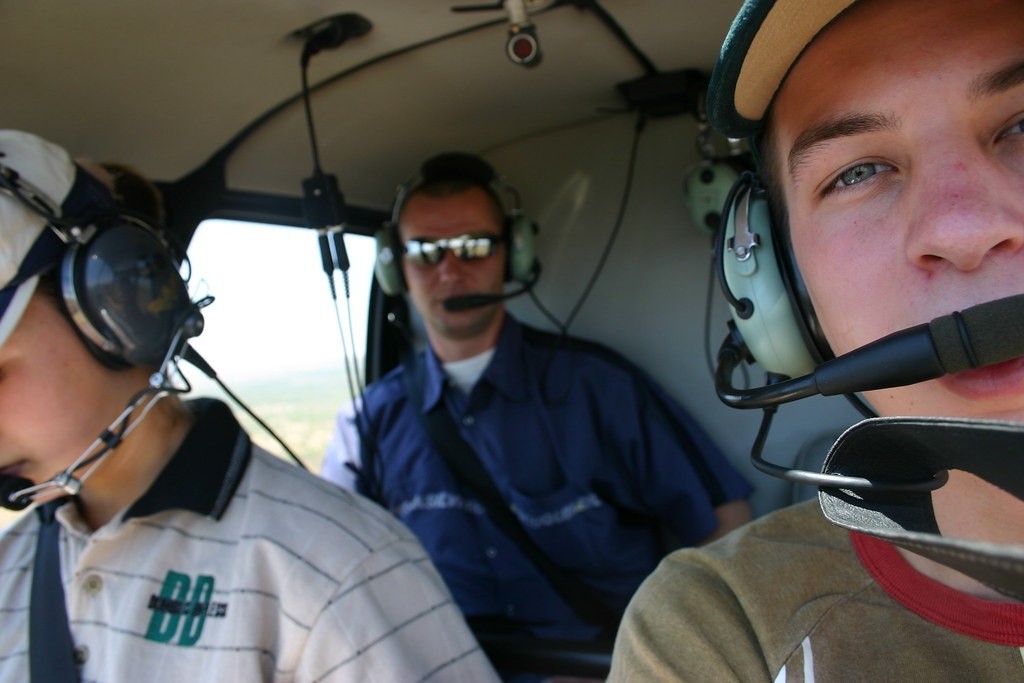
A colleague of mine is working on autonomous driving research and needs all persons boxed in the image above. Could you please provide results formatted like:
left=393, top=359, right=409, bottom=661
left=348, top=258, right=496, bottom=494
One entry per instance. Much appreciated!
left=604, top=1, right=1024, bottom=683
left=314, top=153, right=756, bottom=683
left=0, top=128, right=505, bottom=683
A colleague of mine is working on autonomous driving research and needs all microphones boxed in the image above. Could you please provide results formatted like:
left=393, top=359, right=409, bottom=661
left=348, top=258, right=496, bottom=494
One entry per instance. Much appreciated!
left=715, top=292, right=1024, bottom=408
left=0, top=325, right=190, bottom=511
left=444, top=275, right=539, bottom=312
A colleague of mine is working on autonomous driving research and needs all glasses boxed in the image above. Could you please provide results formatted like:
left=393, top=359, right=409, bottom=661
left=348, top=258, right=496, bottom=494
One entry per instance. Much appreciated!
left=402, top=232, right=504, bottom=264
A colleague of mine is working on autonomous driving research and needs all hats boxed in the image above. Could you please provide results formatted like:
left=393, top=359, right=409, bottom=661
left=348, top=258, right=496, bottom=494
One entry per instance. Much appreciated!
left=704, top=0, right=859, bottom=139
left=1, top=125, right=117, bottom=348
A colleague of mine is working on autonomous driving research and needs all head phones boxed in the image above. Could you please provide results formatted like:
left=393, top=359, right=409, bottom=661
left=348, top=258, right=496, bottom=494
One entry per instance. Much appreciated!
left=0, top=157, right=215, bottom=370
left=373, top=154, right=541, bottom=298
left=702, top=1, right=858, bottom=376
